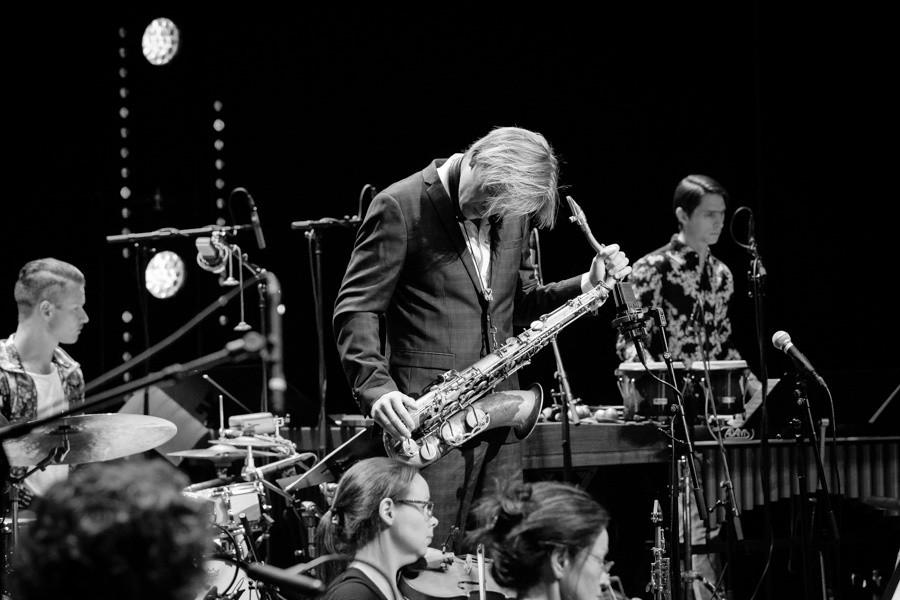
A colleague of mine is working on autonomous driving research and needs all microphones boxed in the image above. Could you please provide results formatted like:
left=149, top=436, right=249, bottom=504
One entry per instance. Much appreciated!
left=612, top=282, right=652, bottom=342
left=196, top=236, right=230, bottom=273
left=771, top=329, right=830, bottom=389
left=247, top=194, right=266, bottom=249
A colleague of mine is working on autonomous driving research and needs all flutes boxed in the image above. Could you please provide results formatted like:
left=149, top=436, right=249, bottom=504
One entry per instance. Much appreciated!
left=646, top=498, right=673, bottom=600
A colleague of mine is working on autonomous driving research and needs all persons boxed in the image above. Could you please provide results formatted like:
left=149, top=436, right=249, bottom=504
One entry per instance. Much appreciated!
left=0, top=258, right=89, bottom=514
left=484, top=479, right=608, bottom=600
left=316, top=457, right=438, bottom=600
left=617, top=174, right=760, bottom=413
left=332, top=127, right=632, bottom=558
left=9, top=455, right=211, bottom=600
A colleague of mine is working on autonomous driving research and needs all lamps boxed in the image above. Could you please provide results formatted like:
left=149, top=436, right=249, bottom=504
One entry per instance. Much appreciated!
left=118, top=237, right=186, bottom=299
left=121, top=11, right=179, bottom=64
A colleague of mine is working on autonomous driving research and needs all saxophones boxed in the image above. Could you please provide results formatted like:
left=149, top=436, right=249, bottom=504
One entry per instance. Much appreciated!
left=382, top=194, right=627, bottom=469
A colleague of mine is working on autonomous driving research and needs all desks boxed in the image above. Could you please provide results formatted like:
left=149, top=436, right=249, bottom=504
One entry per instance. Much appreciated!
left=512, top=418, right=674, bottom=470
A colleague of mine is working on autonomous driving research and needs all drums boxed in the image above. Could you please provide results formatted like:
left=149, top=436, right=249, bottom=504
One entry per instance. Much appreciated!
left=194, top=481, right=265, bottom=539
left=178, top=551, right=263, bottom=600
left=616, top=359, right=749, bottom=422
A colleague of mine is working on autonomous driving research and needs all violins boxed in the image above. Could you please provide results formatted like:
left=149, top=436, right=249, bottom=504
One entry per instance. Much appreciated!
left=398, top=547, right=520, bottom=600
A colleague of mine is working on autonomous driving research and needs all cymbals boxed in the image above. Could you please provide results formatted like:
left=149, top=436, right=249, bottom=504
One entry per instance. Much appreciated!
left=3, top=414, right=178, bottom=469
left=165, top=443, right=284, bottom=462
left=208, top=436, right=284, bottom=447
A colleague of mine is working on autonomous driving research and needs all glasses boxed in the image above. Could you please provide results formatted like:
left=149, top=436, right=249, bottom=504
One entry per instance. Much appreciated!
left=576, top=546, right=614, bottom=573
left=394, top=499, right=433, bottom=519
